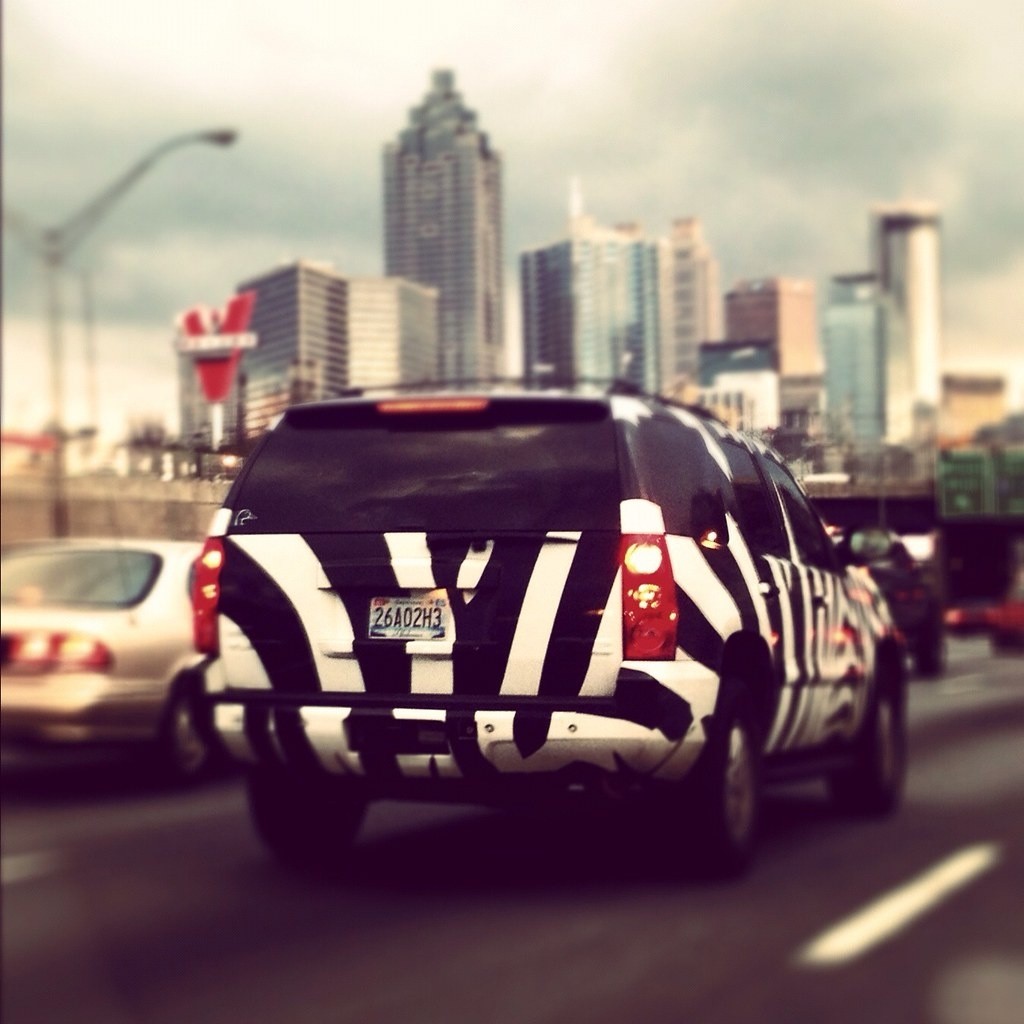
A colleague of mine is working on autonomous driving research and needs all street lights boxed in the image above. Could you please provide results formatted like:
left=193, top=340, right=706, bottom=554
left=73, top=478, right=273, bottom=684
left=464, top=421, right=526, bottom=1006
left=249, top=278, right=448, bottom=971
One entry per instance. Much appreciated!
left=42, top=130, right=237, bottom=532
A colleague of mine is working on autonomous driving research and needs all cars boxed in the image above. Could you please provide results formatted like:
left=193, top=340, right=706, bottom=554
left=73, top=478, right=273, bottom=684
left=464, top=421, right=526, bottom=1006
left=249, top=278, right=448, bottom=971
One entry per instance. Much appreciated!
left=832, top=530, right=949, bottom=676
left=0, top=538, right=225, bottom=791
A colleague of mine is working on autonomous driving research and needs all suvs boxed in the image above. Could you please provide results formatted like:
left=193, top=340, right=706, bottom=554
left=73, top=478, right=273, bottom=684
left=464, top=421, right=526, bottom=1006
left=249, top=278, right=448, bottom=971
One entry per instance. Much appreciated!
left=191, top=378, right=904, bottom=886
